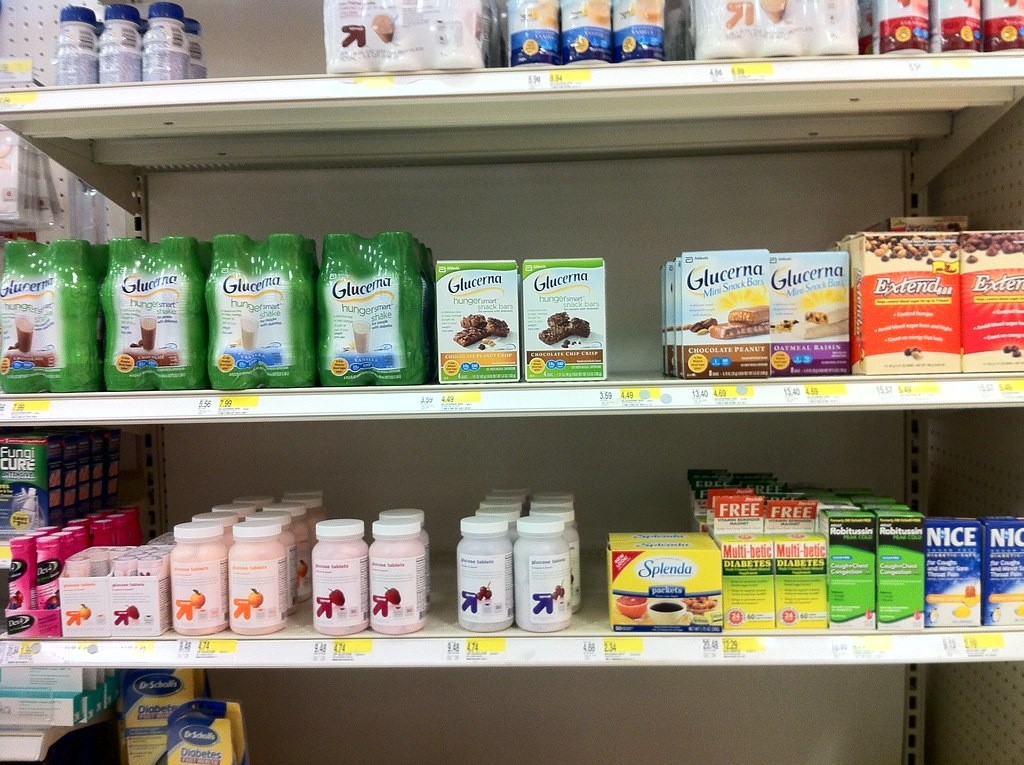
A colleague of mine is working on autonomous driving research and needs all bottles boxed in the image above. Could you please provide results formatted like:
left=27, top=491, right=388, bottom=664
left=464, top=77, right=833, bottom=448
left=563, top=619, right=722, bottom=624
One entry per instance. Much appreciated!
left=170, top=489, right=430, bottom=635
left=858, top=0, right=1024, bottom=53
left=456, top=486, right=582, bottom=633
left=46, top=2, right=209, bottom=84
left=507, top=0, right=667, bottom=67
left=8, top=506, right=166, bottom=641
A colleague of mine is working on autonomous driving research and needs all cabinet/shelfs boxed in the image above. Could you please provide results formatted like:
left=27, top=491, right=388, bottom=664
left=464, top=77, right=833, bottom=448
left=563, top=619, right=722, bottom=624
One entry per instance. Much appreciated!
left=0, top=0, right=1024, bottom=765
